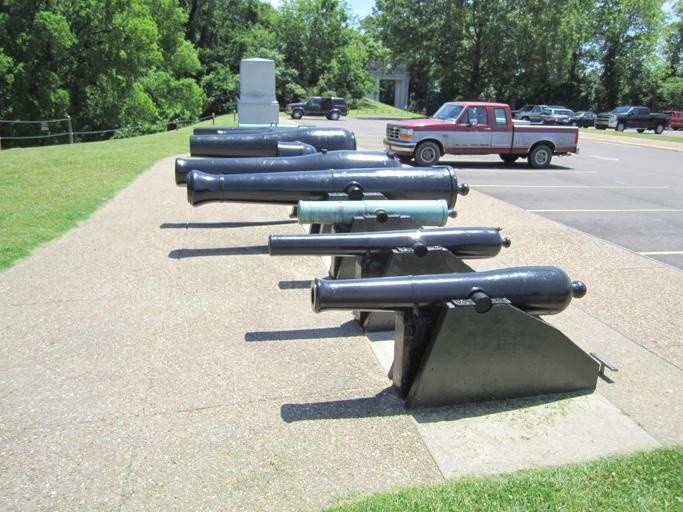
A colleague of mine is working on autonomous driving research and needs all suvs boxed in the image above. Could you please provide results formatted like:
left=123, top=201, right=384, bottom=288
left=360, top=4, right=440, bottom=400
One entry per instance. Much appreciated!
left=286, top=96, right=347, bottom=120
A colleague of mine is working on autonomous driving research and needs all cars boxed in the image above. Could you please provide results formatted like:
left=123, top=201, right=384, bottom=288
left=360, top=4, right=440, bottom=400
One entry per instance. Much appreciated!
left=511, top=104, right=595, bottom=128
left=662, top=111, right=683, bottom=130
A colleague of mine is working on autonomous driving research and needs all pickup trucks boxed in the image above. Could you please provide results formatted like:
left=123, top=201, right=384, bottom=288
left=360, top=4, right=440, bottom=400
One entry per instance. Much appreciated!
left=594, top=106, right=670, bottom=134
left=382, top=101, right=579, bottom=169
